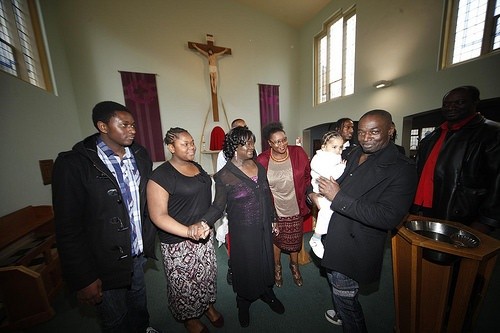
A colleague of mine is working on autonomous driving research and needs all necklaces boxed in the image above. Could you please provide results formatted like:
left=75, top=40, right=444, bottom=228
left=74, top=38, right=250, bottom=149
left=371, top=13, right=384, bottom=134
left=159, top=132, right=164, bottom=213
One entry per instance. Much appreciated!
left=270, top=147, right=289, bottom=162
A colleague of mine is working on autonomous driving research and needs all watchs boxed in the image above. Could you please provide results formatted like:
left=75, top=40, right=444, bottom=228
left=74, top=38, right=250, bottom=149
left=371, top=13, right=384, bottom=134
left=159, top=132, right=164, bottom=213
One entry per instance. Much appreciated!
left=306, top=194, right=314, bottom=206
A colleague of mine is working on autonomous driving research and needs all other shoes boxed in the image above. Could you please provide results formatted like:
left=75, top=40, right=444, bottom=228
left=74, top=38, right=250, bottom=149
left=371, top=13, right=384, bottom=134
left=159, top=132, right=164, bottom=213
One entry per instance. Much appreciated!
left=275, top=265, right=283, bottom=288
left=309, top=237, right=324, bottom=259
left=261, top=294, right=285, bottom=314
left=239, top=305, right=249, bottom=327
left=324, top=310, right=343, bottom=325
left=289, top=263, right=303, bottom=287
left=146, top=327, right=160, bottom=333
left=227, top=268, right=233, bottom=284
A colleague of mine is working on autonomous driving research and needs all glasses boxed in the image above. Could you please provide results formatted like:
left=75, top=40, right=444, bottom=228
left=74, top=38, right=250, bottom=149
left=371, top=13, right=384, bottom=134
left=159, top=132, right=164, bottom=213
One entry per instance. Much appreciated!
left=268, top=137, right=287, bottom=146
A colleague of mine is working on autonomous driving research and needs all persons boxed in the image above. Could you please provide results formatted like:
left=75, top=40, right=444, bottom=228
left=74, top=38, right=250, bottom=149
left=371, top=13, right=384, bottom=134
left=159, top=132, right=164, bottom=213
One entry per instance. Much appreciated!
left=408, top=85, right=500, bottom=240
left=146, top=127, right=224, bottom=333
left=309, top=130, right=347, bottom=259
left=305, top=110, right=418, bottom=333
left=335, top=118, right=358, bottom=162
left=51, top=101, right=159, bottom=333
left=216, top=119, right=247, bottom=174
left=187, top=126, right=285, bottom=328
left=254, top=122, right=311, bottom=288
left=192, top=43, right=228, bottom=93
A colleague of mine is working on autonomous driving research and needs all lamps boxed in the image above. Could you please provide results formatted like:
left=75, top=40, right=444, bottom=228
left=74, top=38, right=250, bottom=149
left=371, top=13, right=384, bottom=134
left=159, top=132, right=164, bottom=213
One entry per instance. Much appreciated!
left=372, top=79, right=393, bottom=89
